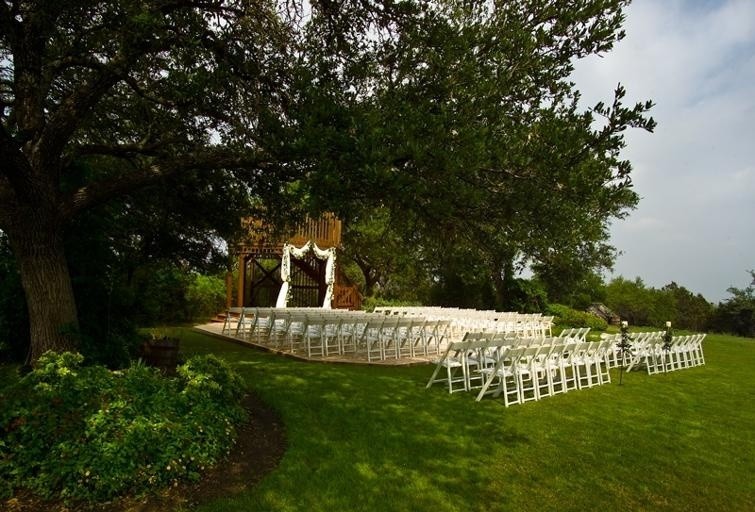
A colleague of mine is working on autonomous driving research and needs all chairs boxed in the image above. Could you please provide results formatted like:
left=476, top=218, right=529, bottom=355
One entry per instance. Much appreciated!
left=425, top=328, right=707, bottom=406
left=221, top=305, right=554, bottom=365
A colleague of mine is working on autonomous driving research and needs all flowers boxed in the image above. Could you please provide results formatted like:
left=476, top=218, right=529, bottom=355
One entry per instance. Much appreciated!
left=616, top=320, right=634, bottom=386
left=661, top=320, right=678, bottom=376
